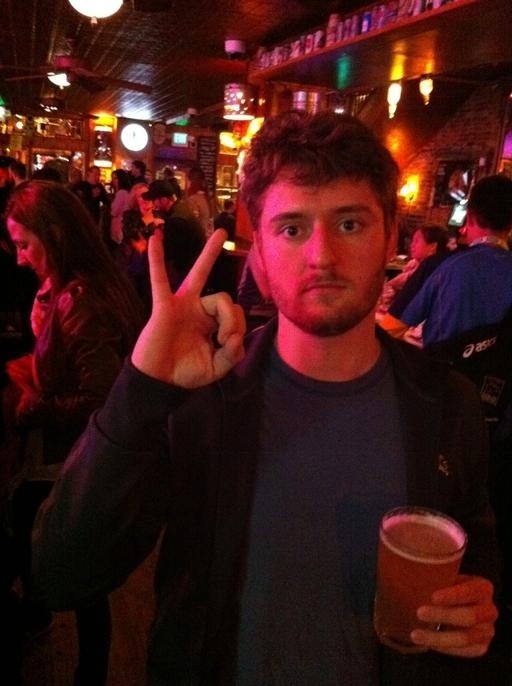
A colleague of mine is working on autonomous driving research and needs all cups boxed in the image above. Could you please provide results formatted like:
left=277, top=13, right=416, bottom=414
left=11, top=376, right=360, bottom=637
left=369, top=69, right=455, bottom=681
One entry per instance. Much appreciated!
left=373, top=505, right=469, bottom=654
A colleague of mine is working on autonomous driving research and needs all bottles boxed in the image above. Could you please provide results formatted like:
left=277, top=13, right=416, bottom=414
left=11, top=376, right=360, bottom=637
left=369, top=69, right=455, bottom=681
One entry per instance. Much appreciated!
left=261, top=1, right=441, bottom=69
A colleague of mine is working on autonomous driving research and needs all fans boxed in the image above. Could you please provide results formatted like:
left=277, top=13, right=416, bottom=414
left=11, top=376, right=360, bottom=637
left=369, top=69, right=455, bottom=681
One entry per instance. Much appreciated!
left=0, top=35, right=152, bottom=95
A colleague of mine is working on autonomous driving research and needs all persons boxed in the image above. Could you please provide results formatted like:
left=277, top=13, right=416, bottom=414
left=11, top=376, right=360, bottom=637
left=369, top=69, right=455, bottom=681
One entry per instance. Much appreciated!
left=0, top=157, right=241, bottom=684
left=31, top=111, right=511, bottom=685
left=380, top=174, right=511, bottom=428
